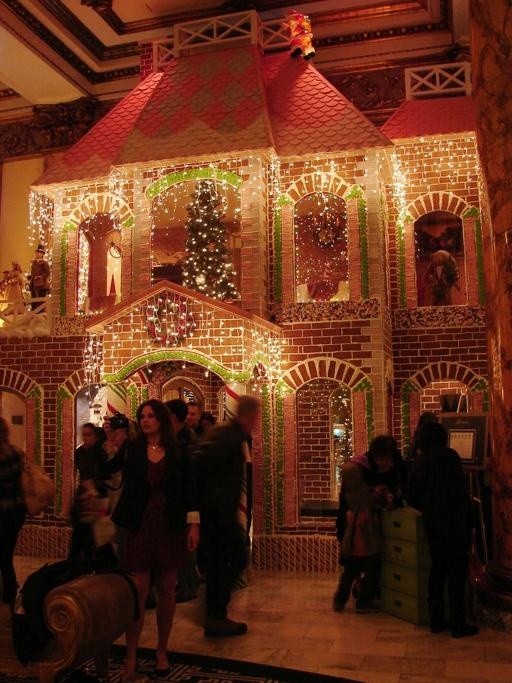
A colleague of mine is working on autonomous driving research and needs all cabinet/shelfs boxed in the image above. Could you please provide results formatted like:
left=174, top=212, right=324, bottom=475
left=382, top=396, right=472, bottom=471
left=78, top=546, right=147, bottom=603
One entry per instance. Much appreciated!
left=379, top=506, right=430, bottom=626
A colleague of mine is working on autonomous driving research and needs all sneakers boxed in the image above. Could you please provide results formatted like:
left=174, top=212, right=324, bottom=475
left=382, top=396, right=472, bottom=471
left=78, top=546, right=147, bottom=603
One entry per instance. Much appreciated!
left=357, top=601, right=379, bottom=614
left=332, top=596, right=344, bottom=611
left=451, top=624, right=479, bottom=637
left=205, top=619, right=248, bottom=636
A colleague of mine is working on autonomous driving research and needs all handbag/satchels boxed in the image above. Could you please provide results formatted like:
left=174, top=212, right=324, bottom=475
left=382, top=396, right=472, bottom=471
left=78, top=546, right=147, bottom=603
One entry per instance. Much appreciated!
left=14, top=447, right=54, bottom=516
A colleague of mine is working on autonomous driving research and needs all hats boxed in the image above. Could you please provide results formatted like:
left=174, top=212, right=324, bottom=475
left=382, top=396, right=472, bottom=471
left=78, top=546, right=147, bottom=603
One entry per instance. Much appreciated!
left=351, top=454, right=370, bottom=470
left=110, top=416, right=128, bottom=428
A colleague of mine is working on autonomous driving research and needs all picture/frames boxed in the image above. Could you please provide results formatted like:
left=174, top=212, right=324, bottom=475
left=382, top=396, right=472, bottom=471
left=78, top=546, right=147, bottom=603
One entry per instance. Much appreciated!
left=447, top=428, right=476, bottom=463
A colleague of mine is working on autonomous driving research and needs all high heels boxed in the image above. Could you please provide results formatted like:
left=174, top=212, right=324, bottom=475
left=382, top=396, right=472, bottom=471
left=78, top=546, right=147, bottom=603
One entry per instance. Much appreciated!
left=154, top=660, right=170, bottom=677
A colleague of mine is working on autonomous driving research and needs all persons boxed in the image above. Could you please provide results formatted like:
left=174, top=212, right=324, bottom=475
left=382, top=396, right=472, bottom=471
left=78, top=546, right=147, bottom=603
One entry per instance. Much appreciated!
left=28, top=245, right=50, bottom=313
left=190, top=396, right=259, bottom=637
left=424, top=251, right=459, bottom=306
left=103, top=399, right=201, bottom=682
left=407, top=423, right=476, bottom=635
left=200, top=412, right=214, bottom=433
left=410, top=411, right=439, bottom=461
left=1, top=415, right=27, bottom=607
left=164, top=399, right=199, bottom=604
left=334, top=462, right=401, bottom=612
left=335, top=436, right=407, bottom=599
left=7, top=259, right=23, bottom=314
left=186, top=402, right=206, bottom=438
left=67, top=424, right=118, bottom=573
